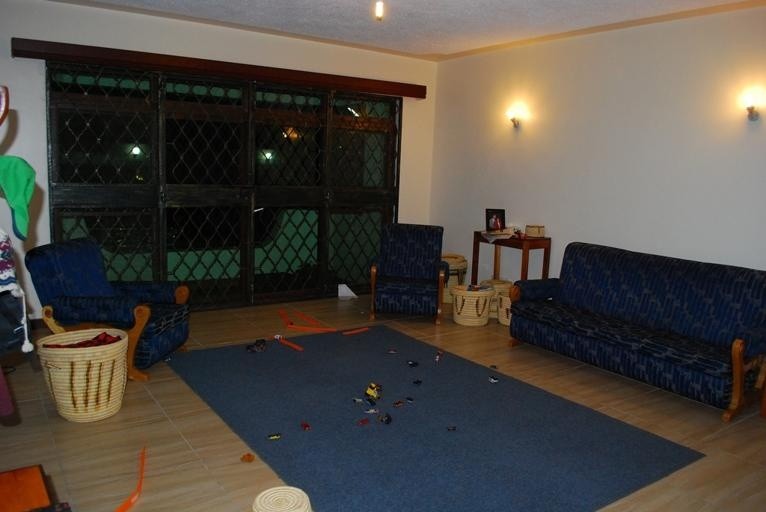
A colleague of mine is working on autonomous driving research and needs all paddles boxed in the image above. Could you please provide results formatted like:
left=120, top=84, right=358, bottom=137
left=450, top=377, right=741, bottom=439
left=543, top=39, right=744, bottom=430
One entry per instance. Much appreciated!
left=164, top=324, right=709, bottom=511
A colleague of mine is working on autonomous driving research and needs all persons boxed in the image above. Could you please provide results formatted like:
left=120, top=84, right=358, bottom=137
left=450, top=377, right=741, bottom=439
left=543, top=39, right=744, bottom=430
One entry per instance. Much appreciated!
left=494, top=218, right=502, bottom=228
left=489, top=214, right=496, bottom=227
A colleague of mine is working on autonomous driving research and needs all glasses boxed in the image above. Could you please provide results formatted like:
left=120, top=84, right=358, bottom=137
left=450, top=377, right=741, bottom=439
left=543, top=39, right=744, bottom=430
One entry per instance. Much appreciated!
left=374, top=0, right=384, bottom=22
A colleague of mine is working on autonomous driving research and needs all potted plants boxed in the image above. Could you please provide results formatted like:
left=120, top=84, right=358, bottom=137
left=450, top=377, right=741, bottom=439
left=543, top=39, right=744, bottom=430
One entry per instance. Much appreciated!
left=485, top=208, right=505, bottom=232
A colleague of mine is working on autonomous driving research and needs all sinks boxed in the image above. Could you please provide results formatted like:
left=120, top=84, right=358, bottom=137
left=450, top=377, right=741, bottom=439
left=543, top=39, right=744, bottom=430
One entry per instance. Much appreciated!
left=510, top=241, right=766, bottom=423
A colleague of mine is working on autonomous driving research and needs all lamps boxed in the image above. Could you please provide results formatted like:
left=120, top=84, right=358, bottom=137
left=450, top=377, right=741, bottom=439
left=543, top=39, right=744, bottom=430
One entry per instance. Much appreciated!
left=25, top=236, right=190, bottom=382
left=370, top=223, right=445, bottom=325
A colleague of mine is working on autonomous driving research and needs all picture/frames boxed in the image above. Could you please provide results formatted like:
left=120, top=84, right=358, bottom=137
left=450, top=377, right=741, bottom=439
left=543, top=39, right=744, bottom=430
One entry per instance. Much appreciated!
left=471, top=231, right=552, bottom=285
